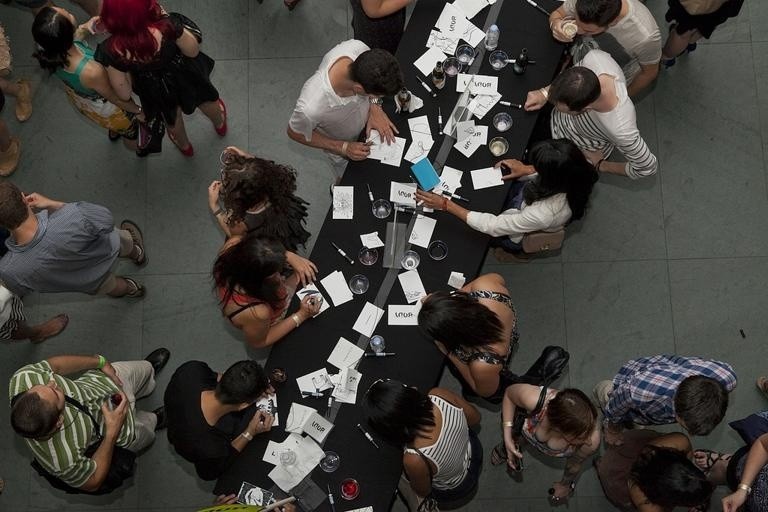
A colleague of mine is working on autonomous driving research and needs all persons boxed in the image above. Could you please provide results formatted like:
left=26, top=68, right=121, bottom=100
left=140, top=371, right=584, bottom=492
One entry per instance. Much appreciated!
left=351, top=1, right=409, bottom=53
left=212, top=234, right=323, bottom=348
left=592, top=354, right=768, bottom=510
left=93, top=0, right=227, bottom=157
left=0, top=285, right=70, bottom=345
left=9, top=348, right=170, bottom=492
left=0, top=27, right=33, bottom=178
left=31, top=6, right=147, bottom=152
left=549, top=1, right=663, bottom=97
left=417, top=272, right=520, bottom=398
left=360, top=377, right=483, bottom=511
left=501, top=383, right=600, bottom=500
left=287, top=39, right=404, bottom=194
left=165, top=359, right=276, bottom=480
left=0, top=179, right=149, bottom=299
left=524, top=48, right=658, bottom=180
left=208, top=147, right=310, bottom=252
left=412, top=138, right=596, bottom=260
left=660, top=1, right=743, bottom=66
left=11, top=0, right=102, bottom=22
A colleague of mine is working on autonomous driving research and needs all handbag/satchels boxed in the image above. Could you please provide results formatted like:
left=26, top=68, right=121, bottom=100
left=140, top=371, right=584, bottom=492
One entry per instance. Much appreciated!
left=522, top=228, right=566, bottom=254
left=137, top=116, right=165, bottom=155
left=461, top=346, right=569, bottom=438
left=30, top=439, right=137, bottom=496
left=166, top=12, right=203, bottom=73
left=397, top=472, right=440, bottom=512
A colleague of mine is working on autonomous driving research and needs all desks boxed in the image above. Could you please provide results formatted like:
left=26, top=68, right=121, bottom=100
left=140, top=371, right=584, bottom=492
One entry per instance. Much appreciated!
left=230, top=1, right=582, bottom=512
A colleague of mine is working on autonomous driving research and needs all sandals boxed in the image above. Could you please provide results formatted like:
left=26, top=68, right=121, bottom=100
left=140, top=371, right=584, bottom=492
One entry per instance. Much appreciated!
left=694, top=449, right=731, bottom=469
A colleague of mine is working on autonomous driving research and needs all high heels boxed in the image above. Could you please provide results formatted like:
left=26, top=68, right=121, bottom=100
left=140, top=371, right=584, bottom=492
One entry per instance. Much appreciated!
left=670, top=24, right=696, bottom=54
left=660, top=56, right=675, bottom=69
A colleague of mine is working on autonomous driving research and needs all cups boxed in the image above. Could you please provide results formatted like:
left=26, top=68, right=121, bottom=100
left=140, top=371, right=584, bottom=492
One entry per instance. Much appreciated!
left=368, top=335, right=384, bottom=353
left=337, top=478, right=360, bottom=501
left=442, top=44, right=508, bottom=78
left=317, top=451, right=340, bottom=473
left=103, top=390, right=123, bottom=412
left=487, top=136, right=508, bottom=157
left=491, top=112, right=513, bottom=132
left=94, top=21, right=107, bottom=36
left=561, top=21, right=577, bottom=39
left=348, top=199, right=449, bottom=295
left=278, top=448, right=297, bottom=469
left=219, top=150, right=236, bottom=167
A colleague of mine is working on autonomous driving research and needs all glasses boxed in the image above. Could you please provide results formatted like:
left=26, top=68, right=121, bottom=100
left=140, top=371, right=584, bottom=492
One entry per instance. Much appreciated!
left=513, top=443, right=523, bottom=473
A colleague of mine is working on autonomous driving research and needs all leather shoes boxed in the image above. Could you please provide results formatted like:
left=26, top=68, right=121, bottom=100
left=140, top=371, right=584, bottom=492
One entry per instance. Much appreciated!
left=144, top=348, right=169, bottom=376
left=152, top=406, right=168, bottom=431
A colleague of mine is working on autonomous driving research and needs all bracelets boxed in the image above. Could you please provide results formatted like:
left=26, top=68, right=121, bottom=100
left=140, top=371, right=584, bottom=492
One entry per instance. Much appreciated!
left=442, top=198, right=448, bottom=212
left=503, top=422, right=514, bottom=426
left=341, top=142, right=348, bottom=157
left=242, top=431, right=253, bottom=440
left=95, top=355, right=106, bottom=371
left=597, top=159, right=603, bottom=170
left=371, top=98, right=383, bottom=104
left=79, top=24, right=84, bottom=32
left=291, top=313, right=302, bottom=327
left=540, top=87, right=548, bottom=100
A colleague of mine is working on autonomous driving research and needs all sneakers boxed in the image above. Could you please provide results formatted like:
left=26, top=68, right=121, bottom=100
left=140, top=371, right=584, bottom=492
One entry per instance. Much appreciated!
left=121, top=220, right=149, bottom=268
left=121, top=275, right=147, bottom=301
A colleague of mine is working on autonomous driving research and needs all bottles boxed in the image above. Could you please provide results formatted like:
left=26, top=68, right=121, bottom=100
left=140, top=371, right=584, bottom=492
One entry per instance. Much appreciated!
left=512, top=47, right=528, bottom=76
left=483, top=24, right=500, bottom=51
left=430, top=60, right=445, bottom=93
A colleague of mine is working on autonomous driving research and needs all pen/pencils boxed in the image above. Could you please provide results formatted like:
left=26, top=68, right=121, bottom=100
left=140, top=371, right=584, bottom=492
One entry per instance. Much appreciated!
left=271, top=498, right=285, bottom=512
left=415, top=76, right=437, bottom=97
left=463, top=51, right=479, bottom=74
left=310, top=298, right=315, bottom=305
left=331, top=242, right=355, bottom=265
left=302, top=392, right=325, bottom=397
left=394, top=207, right=416, bottom=215
left=504, top=59, right=536, bottom=64
left=364, top=352, right=395, bottom=357
left=357, top=424, right=379, bottom=449
left=327, top=397, right=332, bottom=419
left=438, top=107, right=443, bottom=135
left=367, top=184, right=376, bottom=210
left=498, top=100, right=522, bottom=109
left=408, top=174, right=424, bottom=191
left=526, top=0, right=551, bottom=16
left=327, top=484, right=336, bottom=512
left=442, top=191, right=470, bottom=202
left=260, top=415, right=265, bottom=424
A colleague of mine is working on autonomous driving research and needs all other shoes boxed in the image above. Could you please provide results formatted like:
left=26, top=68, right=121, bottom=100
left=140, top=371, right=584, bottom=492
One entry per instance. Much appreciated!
left=31, top=313, right=69, bottom=344
left=167, top=128, right=193, bottom=157
left=136, top=148, right=148, bottom=158
left=0, top=137, right=21, bottom=177
left=15, top=79, right=33, bottom=123
left=214, top=98, right=227, bottom=136
left=108, top=130, right=121, bottom=141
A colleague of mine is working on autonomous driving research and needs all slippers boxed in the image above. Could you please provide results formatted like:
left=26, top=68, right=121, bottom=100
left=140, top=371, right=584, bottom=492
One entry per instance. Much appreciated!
left=491, top=442, right=507, bottom=466
